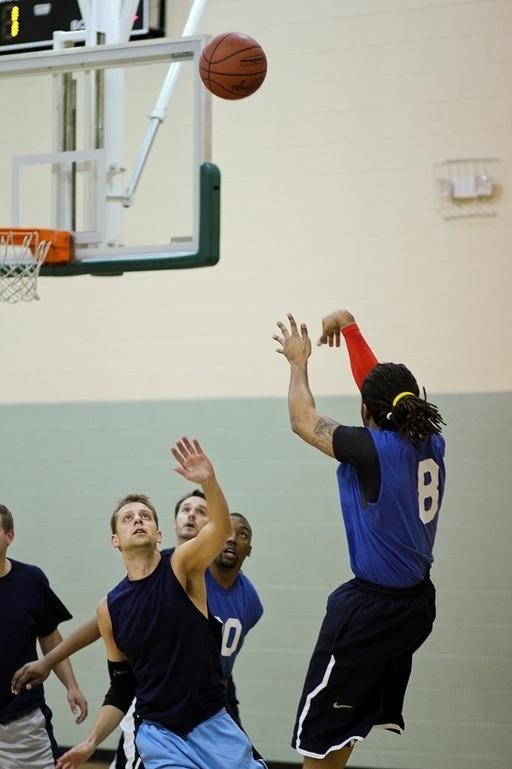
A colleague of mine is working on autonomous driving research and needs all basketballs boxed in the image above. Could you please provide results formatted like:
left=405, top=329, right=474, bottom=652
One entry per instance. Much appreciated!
left=196, top=31, right=268, bottom=100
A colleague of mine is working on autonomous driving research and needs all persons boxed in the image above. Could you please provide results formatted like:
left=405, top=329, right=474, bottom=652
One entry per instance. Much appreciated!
left=271, top=308, right=449, bottom=769
left=56, top=434, right=266, bottom=769
left=0, top=504, right=89, bottom=769
left=160, top=488, right=211, bottom=553
left=10, top=512, right=264, bottom=769
